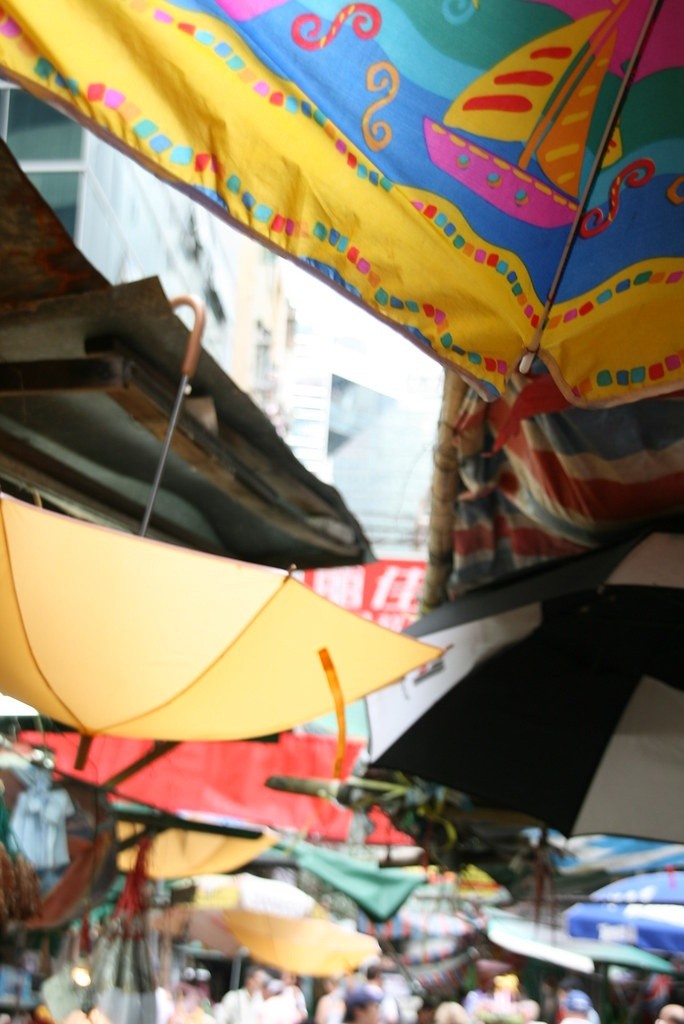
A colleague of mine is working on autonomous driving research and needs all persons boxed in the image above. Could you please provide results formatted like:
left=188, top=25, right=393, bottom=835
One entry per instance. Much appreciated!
left=40, top=955, right=683, bottom=1024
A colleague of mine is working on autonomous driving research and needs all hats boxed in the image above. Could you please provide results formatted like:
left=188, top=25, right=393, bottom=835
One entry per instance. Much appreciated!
left=1, top=965, right=45, bottom=1010
left=561, top=991, right=591, bottom=1013
left=345, top=984, right=383, bottom=1005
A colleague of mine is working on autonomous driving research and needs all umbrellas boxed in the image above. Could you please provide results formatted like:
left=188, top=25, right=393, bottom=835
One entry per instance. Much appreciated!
left=1, top=294, right=456, bottom=769
left=565, top=872, right=683, bottom=954
left=191, top=870, right=379, bottom=974
left=114, top=821, right=276, bottom=884
left=0, top=1, right=684, bottom=416
